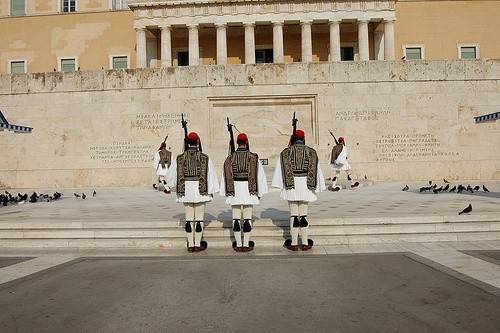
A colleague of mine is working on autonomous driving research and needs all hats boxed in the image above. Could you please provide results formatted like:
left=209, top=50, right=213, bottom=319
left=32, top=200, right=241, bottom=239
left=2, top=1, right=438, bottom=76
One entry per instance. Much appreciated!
left=237, top=133, right=247, bottom=140
left=188, top=132, right=198, bottom=139
left=338, top=137, right=344, bottom=141
left=296, top=130, right=304, bottom=137
left=161, top=143, right=166, bottom=146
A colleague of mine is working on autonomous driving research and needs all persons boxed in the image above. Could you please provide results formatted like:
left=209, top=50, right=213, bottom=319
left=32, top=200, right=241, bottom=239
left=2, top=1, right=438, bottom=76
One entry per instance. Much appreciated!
left=330, top=137, right=359, bottom=191
left=271, top=129, right=326, bottom=251
left=152, top=143, right=173, bottom=194
left=219, top=133, right=269, bottom=252
left=167, top=132, right=219, bottom=252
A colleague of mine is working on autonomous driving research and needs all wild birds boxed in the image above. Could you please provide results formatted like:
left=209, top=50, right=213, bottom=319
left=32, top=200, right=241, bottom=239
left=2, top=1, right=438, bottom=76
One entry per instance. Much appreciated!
left=81, top=193, right=86, bottom=199
left=54, top=68, right=56, bottom=72
left=73, top=193, right=81, bottom=198
left=419, top=178, right=481, bottom=193
left=0, top=190, right=64, bottom=207
left=364, top=174, right=368, bottom=179
left=402, top=184, right=409, bottom=192
left=457, top=203, right=473, bottom=216
left=482, top=184, right=491, bottom=193
left=93, top=190, right=96, bottom=197
left=401, top=56, right=407, bottom=60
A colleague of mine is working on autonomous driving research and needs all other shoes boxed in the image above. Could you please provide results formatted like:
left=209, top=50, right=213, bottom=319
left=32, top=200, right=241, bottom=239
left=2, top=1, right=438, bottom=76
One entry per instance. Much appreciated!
left=153, top=184, right=157, bottom=189
left=233, top=241, right=243, bottom=252
left=164, top=189, right=171, bottom=194
left=351, top=182, right=359, bottom=188
left=331, top=186, right=340, bottom=191
left=187, top=242, right=194, bottom=253
left=302, top=239, right=313, bottom=251
left=194, top=241, right=208, bottom=251
left=242, top=241, right=254, bottom=251
left=284, top=239, right=298, bottom=251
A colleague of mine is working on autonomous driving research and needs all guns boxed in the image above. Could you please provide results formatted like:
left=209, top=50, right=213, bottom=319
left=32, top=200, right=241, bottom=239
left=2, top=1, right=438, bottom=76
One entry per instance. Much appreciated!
left=158, top=133, right=169, bottom=150
left=227, top=117, right=236, bottom=152
left=181, top=113, right=190, bottom=148
left=328, top=128, right=338, bottom=144
left=289, top=110, right=299, bottom=133
